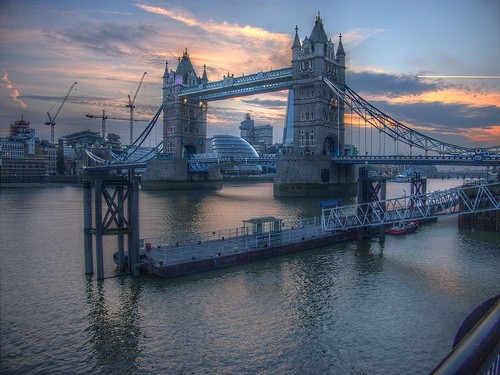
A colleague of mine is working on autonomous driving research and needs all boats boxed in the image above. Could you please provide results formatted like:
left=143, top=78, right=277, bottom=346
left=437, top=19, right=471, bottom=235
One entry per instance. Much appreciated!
left=385, top=222, right=418, bottom=235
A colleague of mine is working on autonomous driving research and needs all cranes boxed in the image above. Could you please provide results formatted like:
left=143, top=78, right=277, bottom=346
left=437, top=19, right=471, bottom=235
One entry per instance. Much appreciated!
left=126, top=71, right=147, bottom=145
left=86, top=108, right=150, bottom=142
left=43, top=81, right=78, bottom=143
